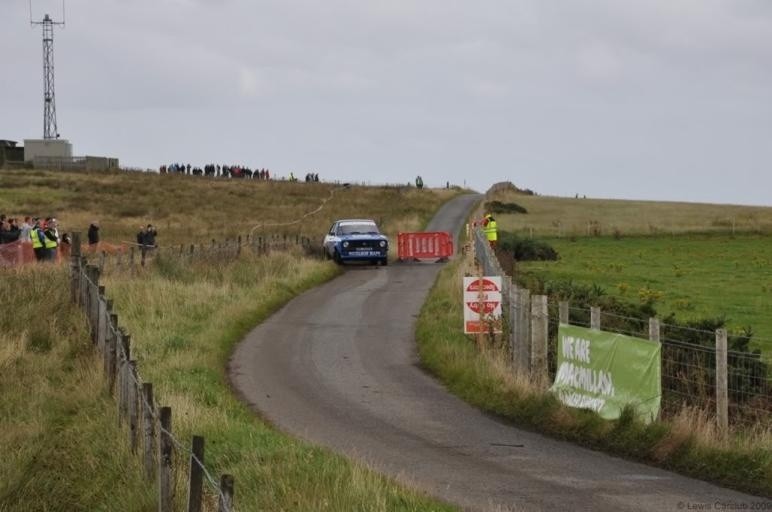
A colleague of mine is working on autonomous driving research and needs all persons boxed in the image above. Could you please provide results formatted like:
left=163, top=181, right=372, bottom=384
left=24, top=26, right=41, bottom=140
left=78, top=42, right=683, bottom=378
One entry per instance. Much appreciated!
left=137, top=225, right=146, bottom=249
left=306, top=173, right=318, bottom=182
left=416, top=176, right=420, bottom=187
left=289, top=173, right=294, bottom=182
left=146, top=224, right=158, bottom=245
left=474, top=212, right=497, bottom=249
left=60, top=233, right=72, bottom=258
left=1, top=212, right=62, bottom=265
left=87, top=220, right=99, bottom=253
left=160, top=163, right=270, bottom=181
left=419, top=177, right=423, bottom=187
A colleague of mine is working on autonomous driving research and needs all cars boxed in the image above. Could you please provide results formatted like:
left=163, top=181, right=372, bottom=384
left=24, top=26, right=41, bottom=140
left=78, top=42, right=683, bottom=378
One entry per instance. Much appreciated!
left=323, top=219, right=388, bottom=267
